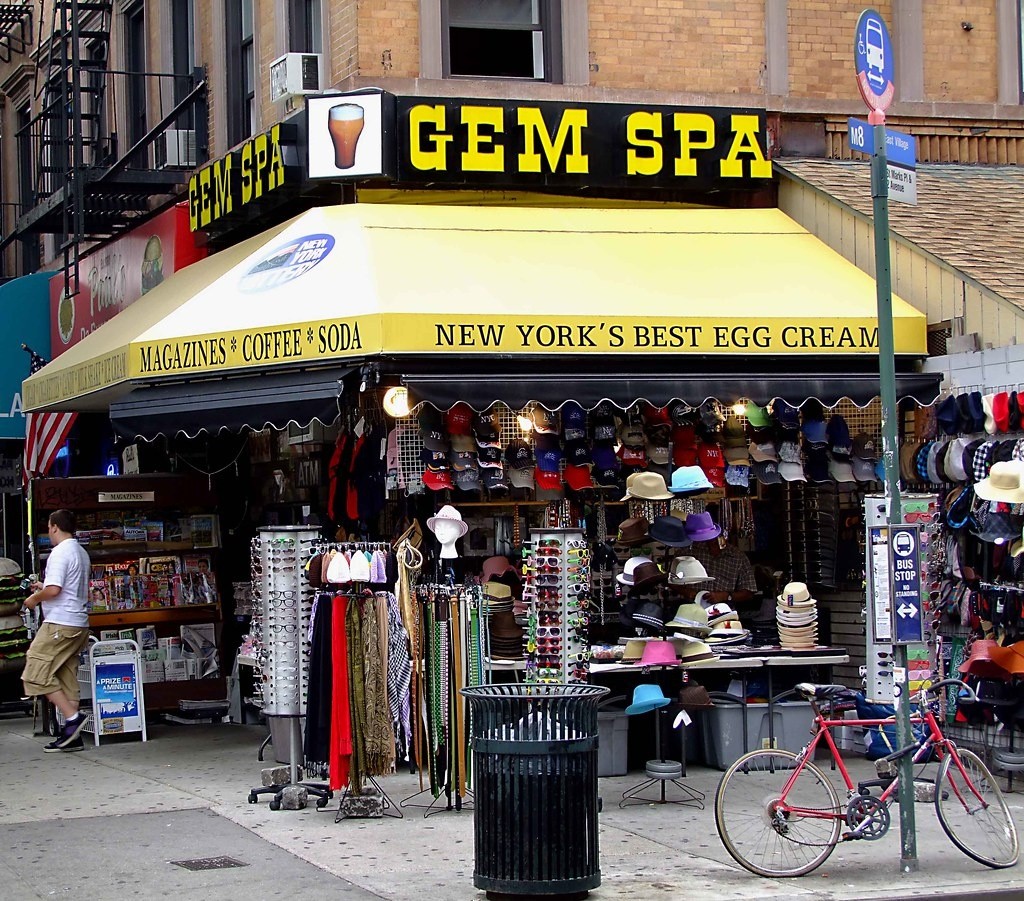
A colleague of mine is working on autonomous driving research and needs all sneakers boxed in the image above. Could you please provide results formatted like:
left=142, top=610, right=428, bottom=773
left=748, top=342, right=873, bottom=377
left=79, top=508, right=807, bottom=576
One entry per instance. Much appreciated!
left=44, top=736, right=84, bottom=753
left=55, top=713, right=89, bottom=748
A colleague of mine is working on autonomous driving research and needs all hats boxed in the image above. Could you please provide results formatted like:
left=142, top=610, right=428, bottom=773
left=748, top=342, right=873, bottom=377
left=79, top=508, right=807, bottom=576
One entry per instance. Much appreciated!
left=426, top=505, right=468, bottom=539
left=415, top=401, right=883, bottom=490
left=900, top=396, right=1024, bottom=729
left=305, top=547, right=399, bottom=589
left=474, top=555, right=525, bottom=660
left=615, top=464, right=822, bottom=716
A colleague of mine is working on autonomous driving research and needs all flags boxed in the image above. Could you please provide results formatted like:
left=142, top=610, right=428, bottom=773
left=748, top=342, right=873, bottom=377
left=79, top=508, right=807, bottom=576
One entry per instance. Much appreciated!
left=22, top=352, right=78, bottom=491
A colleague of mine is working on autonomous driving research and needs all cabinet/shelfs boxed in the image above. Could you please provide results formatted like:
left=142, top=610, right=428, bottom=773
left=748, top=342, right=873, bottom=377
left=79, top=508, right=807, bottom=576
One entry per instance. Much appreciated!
left=40, top=536, right=224, bottom=716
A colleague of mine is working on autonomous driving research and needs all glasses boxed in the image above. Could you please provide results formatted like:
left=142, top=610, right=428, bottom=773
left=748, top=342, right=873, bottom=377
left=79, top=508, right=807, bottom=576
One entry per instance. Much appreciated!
left=596, top=538, right=677, bottom=607
left=250, top=535, right=327, bottom=674
left=859, top=500, right=948, bottom=700
left=521, top=538, right=592, bottom=710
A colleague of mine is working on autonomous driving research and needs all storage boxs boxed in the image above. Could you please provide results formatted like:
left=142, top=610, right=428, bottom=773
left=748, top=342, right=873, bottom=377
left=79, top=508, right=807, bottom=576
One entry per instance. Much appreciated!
left=662, top=699, right=822, bottom=772
left=590, top=703, right=630, bottom=776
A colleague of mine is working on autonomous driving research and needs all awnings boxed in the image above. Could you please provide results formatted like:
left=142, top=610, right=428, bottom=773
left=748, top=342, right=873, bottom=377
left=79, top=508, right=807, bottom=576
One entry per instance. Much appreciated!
left=406, top=372, right=945, bottom=412
left=113, top=399, right=341, bottom=447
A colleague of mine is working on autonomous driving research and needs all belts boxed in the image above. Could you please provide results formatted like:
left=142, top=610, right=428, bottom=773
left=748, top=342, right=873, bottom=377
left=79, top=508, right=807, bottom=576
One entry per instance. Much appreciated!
left=416, top=582, right=487, bottom=800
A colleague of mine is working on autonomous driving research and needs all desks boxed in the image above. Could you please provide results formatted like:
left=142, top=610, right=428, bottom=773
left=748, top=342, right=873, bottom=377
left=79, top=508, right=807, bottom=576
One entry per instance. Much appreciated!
left=487, top=651, right=851, bottom=775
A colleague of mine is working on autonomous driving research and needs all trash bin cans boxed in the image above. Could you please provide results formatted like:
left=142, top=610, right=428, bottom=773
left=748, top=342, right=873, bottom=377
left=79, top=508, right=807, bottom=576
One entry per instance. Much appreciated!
left=458, top=681, right=611, bottom=901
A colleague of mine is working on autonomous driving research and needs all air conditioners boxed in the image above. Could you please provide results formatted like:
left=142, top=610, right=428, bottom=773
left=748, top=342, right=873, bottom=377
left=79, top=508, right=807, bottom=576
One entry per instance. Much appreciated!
left=269, top=52, right=324, bottom=103
left=152, top=129, right=196, bottom=170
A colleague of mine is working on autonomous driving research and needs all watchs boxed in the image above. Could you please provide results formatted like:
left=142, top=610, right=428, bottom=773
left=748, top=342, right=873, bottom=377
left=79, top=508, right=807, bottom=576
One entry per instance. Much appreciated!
left=727, top=592, right=732, bottom=601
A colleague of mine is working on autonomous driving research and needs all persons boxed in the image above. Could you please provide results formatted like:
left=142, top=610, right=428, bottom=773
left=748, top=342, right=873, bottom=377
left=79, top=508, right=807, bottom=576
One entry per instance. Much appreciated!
left=198, top=559, right=209, bottom=573
left=21, top=509, right=90, bottom=754
left=427, top=505, right=468, bottom=558
left=124, top=563, right=138, bottom=575
left=672, top=503, right=757, bottom=607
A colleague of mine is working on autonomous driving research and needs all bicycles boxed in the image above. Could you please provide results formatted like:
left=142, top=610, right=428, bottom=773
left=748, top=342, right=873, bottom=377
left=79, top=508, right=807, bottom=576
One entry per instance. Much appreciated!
left=713, top=679, right=1019, bottom=879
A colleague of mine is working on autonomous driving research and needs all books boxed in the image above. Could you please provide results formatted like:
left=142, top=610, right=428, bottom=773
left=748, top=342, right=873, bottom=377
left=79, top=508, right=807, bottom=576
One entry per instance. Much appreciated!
left=86, top=555, right=216, bottom=613
